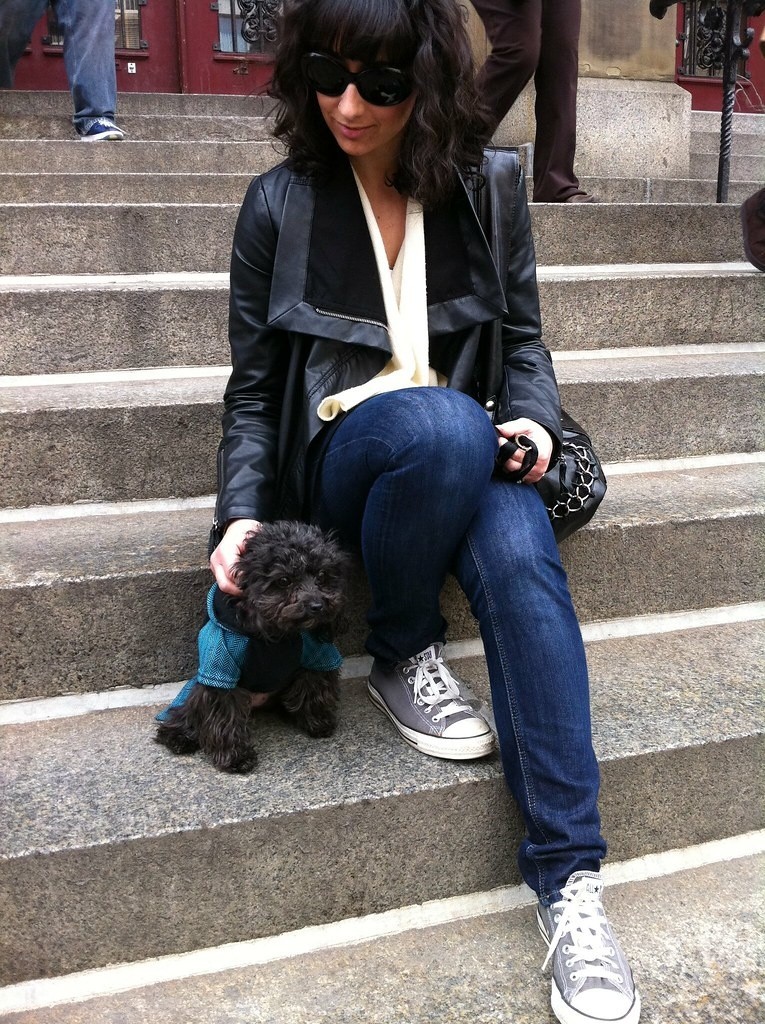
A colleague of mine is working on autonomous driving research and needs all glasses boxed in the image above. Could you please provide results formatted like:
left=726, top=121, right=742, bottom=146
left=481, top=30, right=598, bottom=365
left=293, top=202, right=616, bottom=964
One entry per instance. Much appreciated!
left=295, top=48, right=414, bottom=106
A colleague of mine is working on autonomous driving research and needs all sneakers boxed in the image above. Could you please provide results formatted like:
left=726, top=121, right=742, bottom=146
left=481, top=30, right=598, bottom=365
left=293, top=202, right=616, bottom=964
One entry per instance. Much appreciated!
left=367, top=641, right=496, bottom=760
left=81, top=119, right=130, bottom=142
left=536, top=870, right=641, bottom=1024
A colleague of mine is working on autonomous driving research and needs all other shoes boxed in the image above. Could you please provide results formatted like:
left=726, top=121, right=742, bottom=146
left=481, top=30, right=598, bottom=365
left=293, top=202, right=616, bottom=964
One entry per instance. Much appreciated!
left=740, top=187, right=765, bottom=272
left=565, top=194, right=601, bottom=203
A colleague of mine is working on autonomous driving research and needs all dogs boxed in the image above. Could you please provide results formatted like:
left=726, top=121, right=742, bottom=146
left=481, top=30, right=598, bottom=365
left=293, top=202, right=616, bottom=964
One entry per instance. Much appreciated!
left=152, top=521, right=351, bottom=776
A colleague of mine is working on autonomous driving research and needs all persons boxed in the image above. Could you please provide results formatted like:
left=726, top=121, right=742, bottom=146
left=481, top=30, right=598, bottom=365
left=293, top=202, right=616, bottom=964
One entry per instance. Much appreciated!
left=470, top=0, right=598, bottom=204
left=209, top=0, right=655, bottom=1024
left=0, top=0, right=127, bottom=143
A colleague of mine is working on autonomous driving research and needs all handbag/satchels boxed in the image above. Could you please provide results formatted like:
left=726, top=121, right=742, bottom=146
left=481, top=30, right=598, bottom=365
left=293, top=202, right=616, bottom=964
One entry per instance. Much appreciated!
left=483, top=394, right=607, bottom=545
